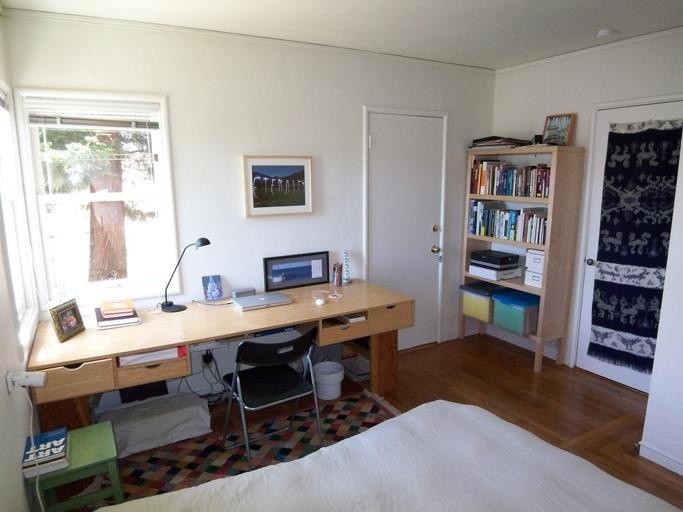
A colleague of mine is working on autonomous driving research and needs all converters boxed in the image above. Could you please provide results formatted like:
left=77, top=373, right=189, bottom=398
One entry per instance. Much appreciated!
left=203, top=353, right=213, bottom=363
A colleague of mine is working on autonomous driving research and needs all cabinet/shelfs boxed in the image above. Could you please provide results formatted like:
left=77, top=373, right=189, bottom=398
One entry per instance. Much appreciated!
left=457, top=142, right=588, bottom=375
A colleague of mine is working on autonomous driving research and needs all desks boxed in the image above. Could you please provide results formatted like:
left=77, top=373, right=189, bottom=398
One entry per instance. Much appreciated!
left=27, top=275, right=417, bottom=512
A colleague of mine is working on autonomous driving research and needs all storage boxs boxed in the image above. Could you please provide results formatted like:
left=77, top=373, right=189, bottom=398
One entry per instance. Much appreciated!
left=460, top=281, right=540, bottom=338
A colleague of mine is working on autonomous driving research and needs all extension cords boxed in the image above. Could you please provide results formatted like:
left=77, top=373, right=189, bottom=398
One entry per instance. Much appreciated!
left=208, top=391, right=230, bottom=402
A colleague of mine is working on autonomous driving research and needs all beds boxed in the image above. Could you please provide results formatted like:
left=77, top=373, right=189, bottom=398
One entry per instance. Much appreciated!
left=96, top=397, right=683, bottom=511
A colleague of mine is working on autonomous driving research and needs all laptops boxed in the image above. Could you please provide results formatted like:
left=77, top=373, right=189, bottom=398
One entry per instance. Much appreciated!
left=231, top=292, right=293, bottom=313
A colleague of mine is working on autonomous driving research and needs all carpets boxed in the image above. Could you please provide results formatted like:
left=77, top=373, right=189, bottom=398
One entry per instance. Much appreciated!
left=70, top=388, right=403, bottom=510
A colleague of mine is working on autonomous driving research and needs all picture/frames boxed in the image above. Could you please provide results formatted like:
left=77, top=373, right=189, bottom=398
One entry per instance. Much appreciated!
left=201, top=272, right=224, bottom=303
left=540, top=112, right=577, bottom=146
left=240, top=151, right=316, bottom=219
left=50, top=297, right=86, bottom=343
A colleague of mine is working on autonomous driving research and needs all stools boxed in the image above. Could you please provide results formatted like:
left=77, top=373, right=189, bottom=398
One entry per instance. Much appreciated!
left=24, top=419, right=125, bottom=512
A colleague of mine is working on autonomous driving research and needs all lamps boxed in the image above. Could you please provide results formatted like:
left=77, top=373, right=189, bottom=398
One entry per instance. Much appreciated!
left=155, top=236, right=211, bottom=313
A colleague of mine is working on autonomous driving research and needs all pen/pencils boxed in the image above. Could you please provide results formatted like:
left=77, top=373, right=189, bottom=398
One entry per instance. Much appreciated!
left=332, top=262, right=342, bottom=287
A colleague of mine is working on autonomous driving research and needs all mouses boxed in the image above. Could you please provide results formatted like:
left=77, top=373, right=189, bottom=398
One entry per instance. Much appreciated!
left=315, top=298, right=325, bottom=306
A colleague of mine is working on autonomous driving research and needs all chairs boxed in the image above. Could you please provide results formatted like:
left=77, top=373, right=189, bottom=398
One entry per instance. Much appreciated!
left=211, top=324, right=323, bottom=469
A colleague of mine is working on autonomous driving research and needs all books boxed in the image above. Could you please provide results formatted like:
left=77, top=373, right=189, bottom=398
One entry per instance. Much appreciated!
left=21, top=426, right=70, bottom=479
left=94, top=298, right=142, bottom=331
left=466, top=158, right=550, bottom=247
